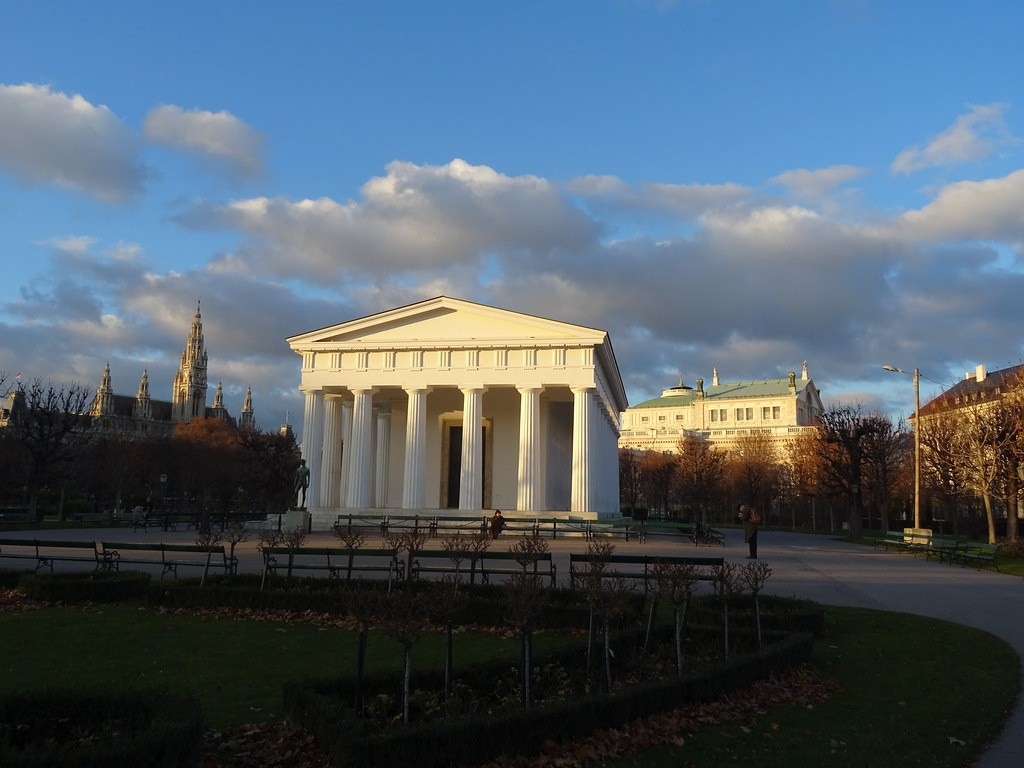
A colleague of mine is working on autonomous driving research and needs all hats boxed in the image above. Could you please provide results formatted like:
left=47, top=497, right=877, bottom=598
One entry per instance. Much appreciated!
left=495, top=510, right=501, bottom=515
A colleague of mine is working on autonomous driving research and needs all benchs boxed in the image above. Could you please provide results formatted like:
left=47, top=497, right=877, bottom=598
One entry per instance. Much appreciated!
left=953, top=541, right=1000, bottom=572
left=483, top=517, right=536, bottom=539
left=408, top=549, right=557, bottom=587
left=0, top=507, right=40, bottom=529
left=588, top=520, right=643, bottom=543
left=334, top=514, right=386, bottom=537
left=536, top=518, right=589, bottom=542
left=874, top=531, right=913, bottom=554
left=0, top=538, right=113, bottom=571
left=263, top=547, right=405, bottom=581
left=899, top=533, right=931, bottom=561
left=569, top=553, right=724, bottom=594
left=102, top=541, right=238, bottom=580
left=925, top=537, right=965, bottom=566
left=432, top=516, right=486, bottom=538
left=644, top=517, right=726, bottom=548
left=79, top=508, right=289, bottom=533
left=383, top=514, right=435, bottom=538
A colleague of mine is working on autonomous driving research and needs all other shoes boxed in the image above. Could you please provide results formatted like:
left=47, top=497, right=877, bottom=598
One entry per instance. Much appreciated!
left=492, top=537, right=497, bottom=539
left=744, top=556, right=757, bottom=559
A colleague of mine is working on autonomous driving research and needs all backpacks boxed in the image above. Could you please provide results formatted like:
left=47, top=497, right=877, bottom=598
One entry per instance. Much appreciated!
left=748, top=508, right=760, bottom=523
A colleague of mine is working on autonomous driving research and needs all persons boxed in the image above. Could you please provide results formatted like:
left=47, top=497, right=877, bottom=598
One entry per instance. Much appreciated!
left=293, top=459, right=310, bottom=507
left=490, top=509, right=505, bottom=539
left=738, top=502, right=758, bottom=559
